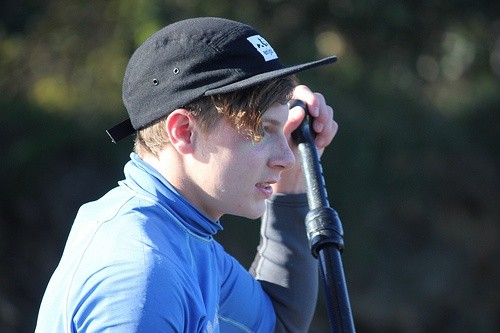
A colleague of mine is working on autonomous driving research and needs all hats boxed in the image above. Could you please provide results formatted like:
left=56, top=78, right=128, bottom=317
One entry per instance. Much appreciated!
left=105, top=17, right=338, bottom=144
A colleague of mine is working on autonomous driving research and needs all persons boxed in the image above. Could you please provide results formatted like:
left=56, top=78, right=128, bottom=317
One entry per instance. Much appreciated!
left=33, top=18, right=338, bottom=333
